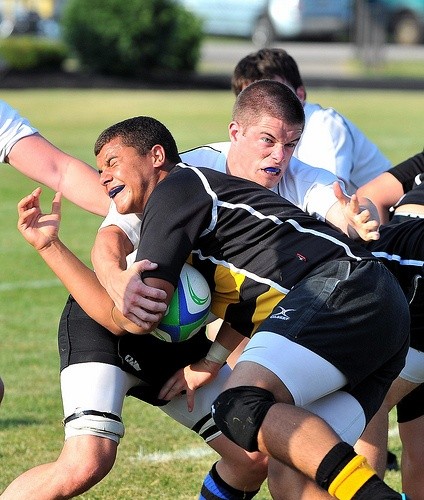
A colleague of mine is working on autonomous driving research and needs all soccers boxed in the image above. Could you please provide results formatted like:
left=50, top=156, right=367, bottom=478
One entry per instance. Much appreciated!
left=150, top=263, right=211, bottom=343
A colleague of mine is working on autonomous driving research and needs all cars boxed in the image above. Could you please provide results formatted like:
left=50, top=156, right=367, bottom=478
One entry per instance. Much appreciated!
left=182, top=0, right=424, bottom=51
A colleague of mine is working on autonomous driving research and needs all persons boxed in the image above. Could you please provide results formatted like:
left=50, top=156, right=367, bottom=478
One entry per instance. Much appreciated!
left=0, top=47, right=424, bottom=500
